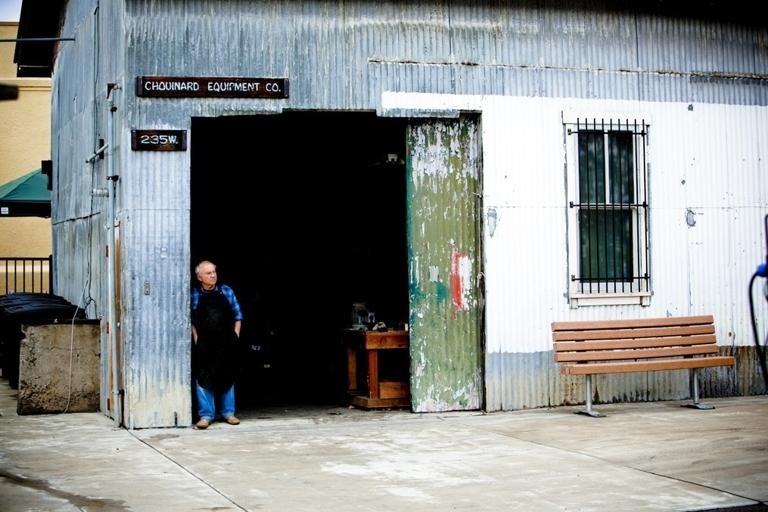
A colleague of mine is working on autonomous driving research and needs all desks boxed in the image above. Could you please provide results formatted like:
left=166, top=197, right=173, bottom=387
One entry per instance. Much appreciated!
left=340, top=329, right=411, bottom=409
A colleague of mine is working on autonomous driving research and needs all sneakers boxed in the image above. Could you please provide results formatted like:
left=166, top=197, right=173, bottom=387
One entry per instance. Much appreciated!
left=224, top=415, right=240, bottom=425
left=195, top=417, right=210, bottom=430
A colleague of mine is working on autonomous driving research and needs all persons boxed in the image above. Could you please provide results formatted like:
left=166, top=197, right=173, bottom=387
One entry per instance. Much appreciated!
left=191, top=261, right=245, bottom=430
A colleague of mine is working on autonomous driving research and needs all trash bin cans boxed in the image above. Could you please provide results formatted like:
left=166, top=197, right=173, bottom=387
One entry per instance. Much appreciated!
left=0, top=292, right=86, bottom=391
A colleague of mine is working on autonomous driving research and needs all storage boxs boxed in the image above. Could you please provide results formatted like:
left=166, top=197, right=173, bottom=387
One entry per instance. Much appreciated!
left=366, top=381, right=410, bottom=400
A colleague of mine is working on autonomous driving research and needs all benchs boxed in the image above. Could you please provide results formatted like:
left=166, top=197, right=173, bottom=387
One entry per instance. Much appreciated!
left=550, top=314, right=735, bottom=416
